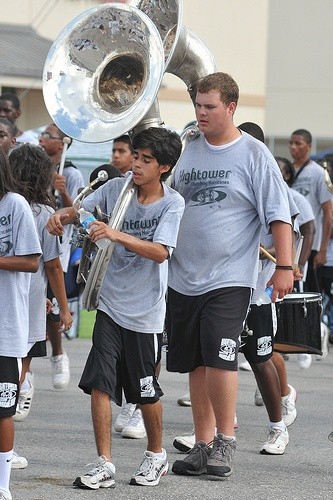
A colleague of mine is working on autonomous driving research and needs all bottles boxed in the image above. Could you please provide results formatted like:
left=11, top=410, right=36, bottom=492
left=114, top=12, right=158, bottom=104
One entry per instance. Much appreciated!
left=256, top=283, right=279, bottom=306
left=77, top=207, right=111, bottom=249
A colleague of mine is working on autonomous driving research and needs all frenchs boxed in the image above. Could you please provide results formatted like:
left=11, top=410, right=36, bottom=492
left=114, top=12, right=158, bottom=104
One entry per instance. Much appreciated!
left=39, top=0, right=168, bottom=316
left=139, top=2, right=222, bottom=110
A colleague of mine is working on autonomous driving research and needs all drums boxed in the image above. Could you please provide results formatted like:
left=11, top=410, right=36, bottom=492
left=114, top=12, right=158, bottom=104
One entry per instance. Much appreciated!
left=271, top=291, right=327, bottom=357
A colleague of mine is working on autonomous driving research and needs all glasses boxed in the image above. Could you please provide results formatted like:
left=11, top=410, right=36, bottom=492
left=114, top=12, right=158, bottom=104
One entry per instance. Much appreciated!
left=39, top=132, right=63, bottom=141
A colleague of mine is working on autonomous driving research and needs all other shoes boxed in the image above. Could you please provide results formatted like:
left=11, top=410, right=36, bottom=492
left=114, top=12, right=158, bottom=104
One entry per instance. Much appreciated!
left=177, top=397, right=192, bottom=407
left=10, top=452, right=28, bottom=469
left=254, top=386, right=264, bottom=406
left=238, top=360, right=252, bottom=371
left=296, top=352, right=312, bottom=370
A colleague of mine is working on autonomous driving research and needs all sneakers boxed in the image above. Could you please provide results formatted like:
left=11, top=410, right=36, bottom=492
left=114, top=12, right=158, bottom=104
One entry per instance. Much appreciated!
left=129, top=449, right=169, bottom=485
left=259, top=424, right=290, bottom=455
left=172, top=440, right=212, bottom=476
left=173, top=427, right=218, bottom=453
left=73, top=455, right=117, bottom=490
left=50, top=351, right=70, bottom=389
left=121, top=408, right=147, bottom=439
left=12, top=380, right=34, bottom=421
left=205, top=433, right=237, bottom=480
left=192, top=410, right=238, bottom=431
left=280, top=384, right=297, bottom=427
left=113, top=398, right=137, bottom=436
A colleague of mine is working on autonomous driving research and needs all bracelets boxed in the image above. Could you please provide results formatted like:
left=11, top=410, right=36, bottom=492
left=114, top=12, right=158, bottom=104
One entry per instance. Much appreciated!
left=275, top=266, right=293, bottom=270
left=298, top=266, right=304, bottom=271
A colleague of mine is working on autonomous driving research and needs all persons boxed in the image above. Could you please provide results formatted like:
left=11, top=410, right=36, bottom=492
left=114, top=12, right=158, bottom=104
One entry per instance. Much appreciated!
left=0, top=92, right=333, bottom=467
left=45, top=127, right=186, bottom=489
left=164, top=72, right=293, bottom=476
left=0, top=144, right=42, bottom=500
